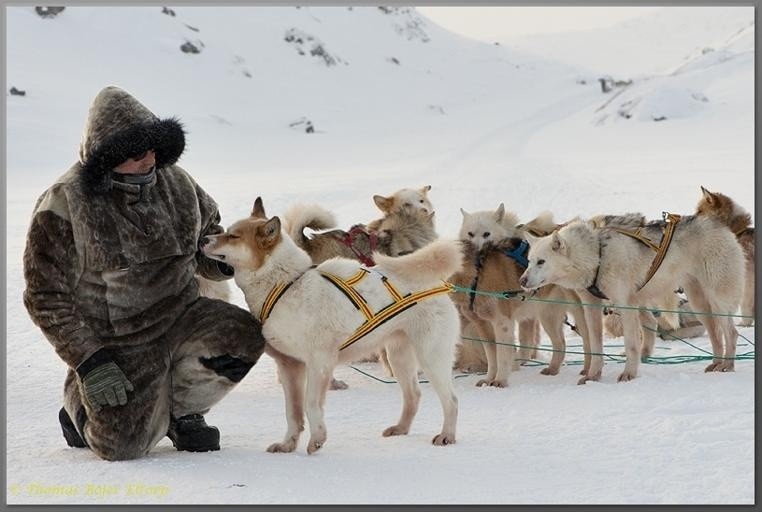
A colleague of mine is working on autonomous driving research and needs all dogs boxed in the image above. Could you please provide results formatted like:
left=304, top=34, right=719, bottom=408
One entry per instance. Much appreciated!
left=198, top=195, right=463, bottom=454
left=372, top=182, right=434, bottom=214
left=447, top=185, right=757, bottom=389
left=279, top=201, right=439, bottom=392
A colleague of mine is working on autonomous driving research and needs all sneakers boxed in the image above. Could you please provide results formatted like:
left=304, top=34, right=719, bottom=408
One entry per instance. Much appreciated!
left=55, top=405, right=83, bottom=449
left=166, top=412, right=224, bottom=456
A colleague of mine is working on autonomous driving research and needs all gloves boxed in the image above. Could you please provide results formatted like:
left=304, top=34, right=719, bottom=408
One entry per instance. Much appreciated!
left=79, top=363, right=138, bottom=414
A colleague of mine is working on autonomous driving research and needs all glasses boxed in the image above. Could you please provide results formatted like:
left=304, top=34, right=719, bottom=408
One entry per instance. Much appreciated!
left=128, top=148, right=155, bottom=161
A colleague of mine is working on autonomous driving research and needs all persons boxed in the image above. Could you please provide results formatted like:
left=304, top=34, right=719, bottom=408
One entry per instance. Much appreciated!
left=23, top=86, right=265, bottom=461
